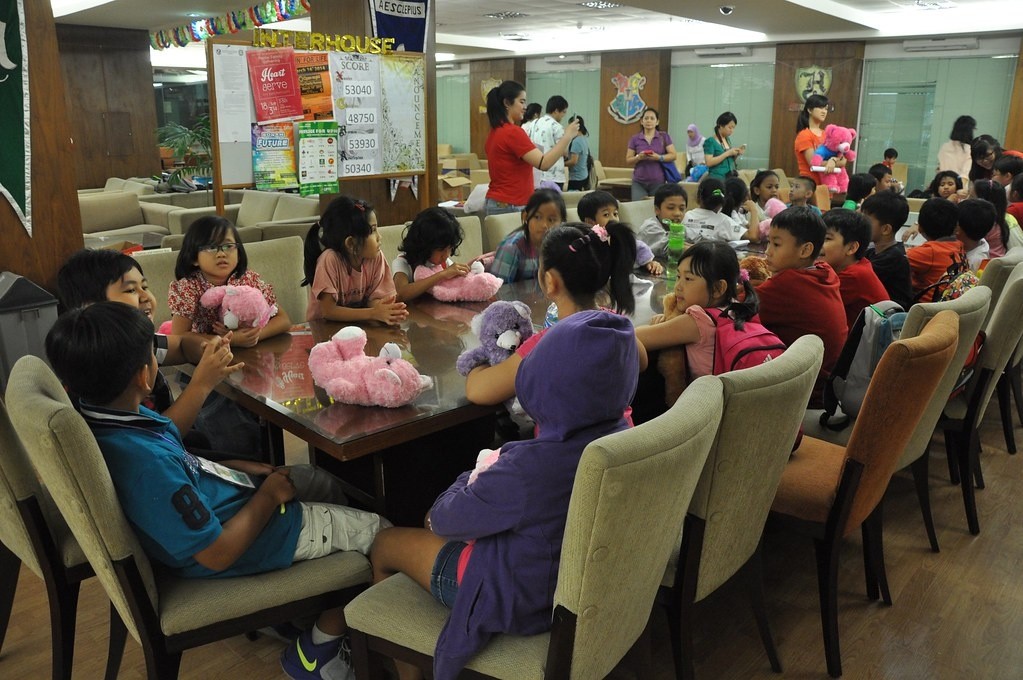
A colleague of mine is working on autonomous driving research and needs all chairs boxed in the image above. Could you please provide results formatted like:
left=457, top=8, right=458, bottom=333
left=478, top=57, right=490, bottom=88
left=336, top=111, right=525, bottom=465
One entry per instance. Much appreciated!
left=344, top=373, right=723, bottom=680
left=790, top=279, right=1000, bottom=601
left=907, top=197, right=928, bottom=212
left=655, top=329, right=830, bottom=680
left=379, top=224, right=410, bottom=261
left=483, top=211, right=524, bottom=252
left=772, top=310, right=963, bottom=680
left=0, top=393, right=131, bottom=680
left=619, top=194, right=656, bottom=225
left=132, top=235, right=310, bottom=350
left=407, top=214, right=487, bottom=287
left=977, top=246, right=1023, bottom=328
left=951, top=261, right=1023, bottom=537
left=5, top=354, right=373, bottom=680
left=566, top=205, right=582, bottom=223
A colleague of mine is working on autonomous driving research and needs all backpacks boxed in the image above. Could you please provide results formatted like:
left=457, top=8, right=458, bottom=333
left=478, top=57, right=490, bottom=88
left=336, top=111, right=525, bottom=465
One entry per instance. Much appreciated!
left=703, top=304, right=805, bottom=452
left=911, top=253, right=989, bottom=398
left=819, top=299, right=909, bottom=432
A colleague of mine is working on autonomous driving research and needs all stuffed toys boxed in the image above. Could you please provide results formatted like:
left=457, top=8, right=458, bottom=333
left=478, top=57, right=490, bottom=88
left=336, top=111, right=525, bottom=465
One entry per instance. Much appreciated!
left=738, top=254, right=769, bottom=287
left=632, top=238, right=654, bottom=272
left=455, top=300, right=533, bottom=378
left=313, top=401, right=431, bottom=441
left=759, top=198, right=787, bottom=240
left=413, top=261, right=504, bottom=302
left=657, top=291, right=686, bottom=409
left=810, top=124, right=857, bottom=194
left=201, top=285, right=271, bottom=330
left=308, top=327, right=432, bottom=407
left=428, top=448, right=502, bottom=545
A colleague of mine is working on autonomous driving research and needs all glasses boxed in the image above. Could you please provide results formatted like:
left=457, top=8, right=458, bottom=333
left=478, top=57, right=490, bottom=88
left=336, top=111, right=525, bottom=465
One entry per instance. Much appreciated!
left=195, top=242, right=238, bottom=254
left=976, top=149, right=994, bottom=164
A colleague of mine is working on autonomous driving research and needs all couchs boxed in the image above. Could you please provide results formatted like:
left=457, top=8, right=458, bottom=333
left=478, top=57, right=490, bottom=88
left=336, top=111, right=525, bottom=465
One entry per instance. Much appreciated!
left=75, top=174, right=321, bottom=251
left=594, top=161, right=634, bottom=190
left=738, top=167, right=794, bottom=189
left=452, top=152, right=491, bottom=192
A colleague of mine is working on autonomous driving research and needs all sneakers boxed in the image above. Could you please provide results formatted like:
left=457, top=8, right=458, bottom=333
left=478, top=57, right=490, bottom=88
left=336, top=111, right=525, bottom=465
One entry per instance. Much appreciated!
left=278, top=629, right=355, bottom=680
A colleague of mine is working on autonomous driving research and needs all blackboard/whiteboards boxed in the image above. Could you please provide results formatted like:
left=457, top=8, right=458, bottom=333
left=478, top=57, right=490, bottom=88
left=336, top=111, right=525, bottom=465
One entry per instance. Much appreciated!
left=205, top=36, right=443, bottom=188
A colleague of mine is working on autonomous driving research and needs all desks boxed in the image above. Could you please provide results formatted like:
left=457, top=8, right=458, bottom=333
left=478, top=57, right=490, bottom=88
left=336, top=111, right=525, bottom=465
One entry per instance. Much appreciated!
left=437, top=201, right=466, bottom=216
left=599, top=178, right=631, bottom=198
left=180, top=234, right=780, bottom=506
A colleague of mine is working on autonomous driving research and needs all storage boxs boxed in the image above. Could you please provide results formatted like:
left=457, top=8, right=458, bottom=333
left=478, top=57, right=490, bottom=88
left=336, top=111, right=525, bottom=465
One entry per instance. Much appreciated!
left=443, top=159, right=471, bottom=177
left=438, top=173, right=472, bottom=202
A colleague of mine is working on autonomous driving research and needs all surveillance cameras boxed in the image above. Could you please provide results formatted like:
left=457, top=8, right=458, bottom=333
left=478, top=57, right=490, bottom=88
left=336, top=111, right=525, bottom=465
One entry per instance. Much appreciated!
left=720, top=6, right=732, bottom=15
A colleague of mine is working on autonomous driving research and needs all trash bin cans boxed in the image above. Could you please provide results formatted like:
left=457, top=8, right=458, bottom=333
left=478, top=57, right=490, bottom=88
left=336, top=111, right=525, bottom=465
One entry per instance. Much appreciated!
left=0, top=271, right=59, bottom=501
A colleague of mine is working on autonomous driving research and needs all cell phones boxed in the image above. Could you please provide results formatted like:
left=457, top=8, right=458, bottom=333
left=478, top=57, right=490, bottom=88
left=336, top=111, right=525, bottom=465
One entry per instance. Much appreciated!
left=574, top=112, right=577, bottom=121
left=738, top=144, right=746, bottom=150
left=644, top=150, right=653, bottom=156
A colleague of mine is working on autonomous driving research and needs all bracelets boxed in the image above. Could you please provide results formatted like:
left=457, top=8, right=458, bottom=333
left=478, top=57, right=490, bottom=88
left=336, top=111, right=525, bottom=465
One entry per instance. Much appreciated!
left=831, top=156, right=837, bottom=164
left=659, top=155, right=664, bottom=163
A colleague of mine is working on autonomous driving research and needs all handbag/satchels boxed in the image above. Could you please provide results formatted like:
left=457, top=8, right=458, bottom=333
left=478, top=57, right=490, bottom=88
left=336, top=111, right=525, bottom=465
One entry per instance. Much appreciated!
left=663, top=161, right=681, bottom=182
left=585, top=148, right=598, bottom=190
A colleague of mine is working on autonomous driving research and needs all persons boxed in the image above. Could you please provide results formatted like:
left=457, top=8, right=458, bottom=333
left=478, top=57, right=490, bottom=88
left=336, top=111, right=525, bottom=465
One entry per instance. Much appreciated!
left=751, top=115, right=1023, bottom=406
left=211, top=331, right=291, bottom=408
left=39, top=197, right=393, bottom=680
left=376, top=177, right=758, bottom=680
left=484, top=81, right=589, bottom=217
left=794, top=94, right=830, bottom=185
left=623, top=108, right=745, bottom=205
left=637, top=269, right=667, bottom=315
left=392, top=304, right=465, bottom=374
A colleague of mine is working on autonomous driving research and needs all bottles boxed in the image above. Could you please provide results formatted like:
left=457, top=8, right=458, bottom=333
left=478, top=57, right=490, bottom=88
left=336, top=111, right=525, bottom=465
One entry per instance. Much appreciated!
left=542, top=302, right=558, bottom=330
left=662, top=219, right=684, bottom=278
